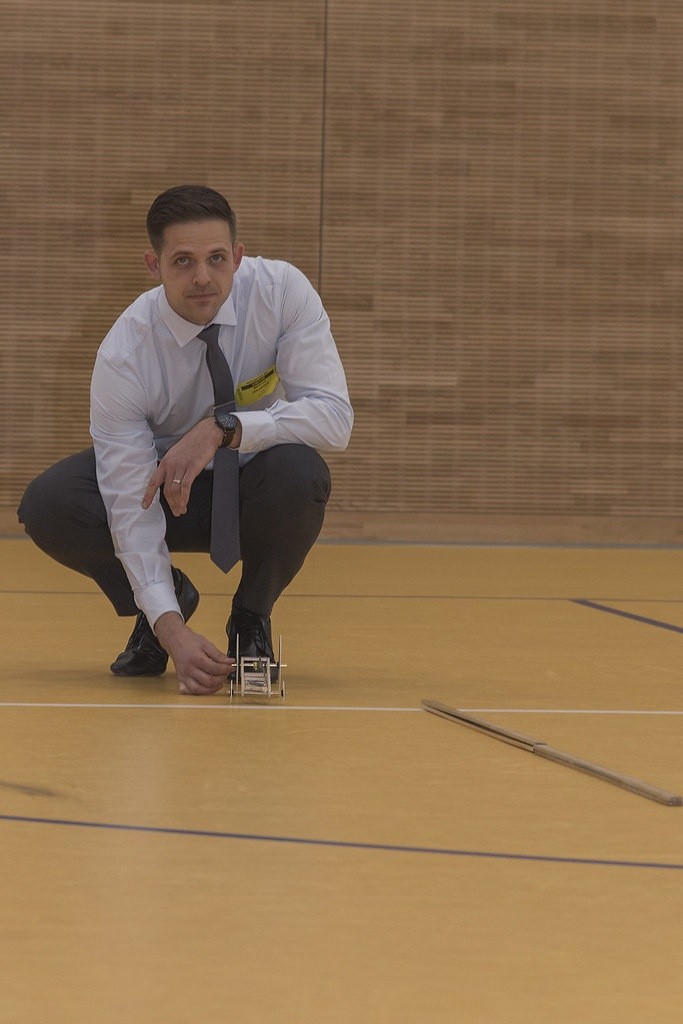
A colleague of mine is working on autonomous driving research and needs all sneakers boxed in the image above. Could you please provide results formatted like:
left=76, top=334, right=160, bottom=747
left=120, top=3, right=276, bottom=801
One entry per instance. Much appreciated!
left=110, top=567, right=200, bottom=678
left=225, top=596, right=277, bottom=685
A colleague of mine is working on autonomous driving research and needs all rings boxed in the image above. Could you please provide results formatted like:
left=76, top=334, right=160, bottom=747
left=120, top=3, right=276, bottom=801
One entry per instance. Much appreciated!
left=172, top=479, right=181, bottom=484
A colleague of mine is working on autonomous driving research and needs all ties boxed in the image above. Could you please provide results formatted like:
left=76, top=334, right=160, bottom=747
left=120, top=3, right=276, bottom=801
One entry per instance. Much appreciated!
left=195, top=323, right=240, bottom=573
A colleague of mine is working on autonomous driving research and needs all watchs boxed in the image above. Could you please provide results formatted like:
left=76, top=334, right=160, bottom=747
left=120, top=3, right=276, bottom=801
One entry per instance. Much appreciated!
left=214, top=414, right=237, bottom=446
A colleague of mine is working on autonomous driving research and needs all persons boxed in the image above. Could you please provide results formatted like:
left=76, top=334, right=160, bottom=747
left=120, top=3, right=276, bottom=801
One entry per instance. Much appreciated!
left=18, top=186, right=353, bottom=694
